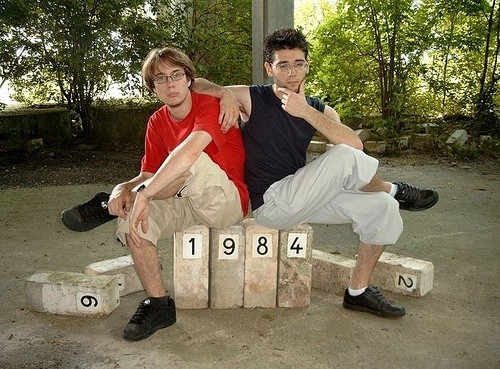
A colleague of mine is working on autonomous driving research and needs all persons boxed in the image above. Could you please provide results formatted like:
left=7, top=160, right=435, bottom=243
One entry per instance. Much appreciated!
left=190, top=28, right=439, bottom=318
left=60, top=47, right=248, bottom=341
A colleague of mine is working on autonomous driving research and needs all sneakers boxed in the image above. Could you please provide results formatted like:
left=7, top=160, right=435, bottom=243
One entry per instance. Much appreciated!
left=391, top=180, right=439, bottom=213
left=122, top=296, right=177, bottom=342
left=343, top=286, right=405, bottom=318
left=60, top=192, right=121, bottom=231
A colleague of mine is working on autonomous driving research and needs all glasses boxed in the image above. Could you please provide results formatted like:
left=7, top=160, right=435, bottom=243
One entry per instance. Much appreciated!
left=269, top=62, right=307, bottom=72
left=152, top=71, right=187, bottom=84
